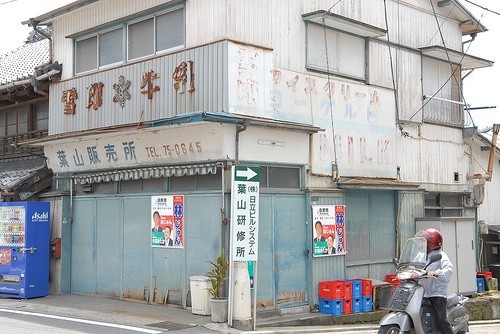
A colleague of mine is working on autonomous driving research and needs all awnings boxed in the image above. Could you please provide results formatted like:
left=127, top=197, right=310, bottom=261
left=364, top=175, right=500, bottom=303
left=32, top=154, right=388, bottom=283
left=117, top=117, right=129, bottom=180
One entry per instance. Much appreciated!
left=301, top=9, right=388, bottom=40
left=419, top=45, right=493, bottom=79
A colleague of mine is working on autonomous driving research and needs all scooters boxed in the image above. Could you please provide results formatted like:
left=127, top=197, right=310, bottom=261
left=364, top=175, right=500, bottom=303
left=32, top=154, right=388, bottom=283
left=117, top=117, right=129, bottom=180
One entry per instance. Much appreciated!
left=377, top=237, right=469, bottom=334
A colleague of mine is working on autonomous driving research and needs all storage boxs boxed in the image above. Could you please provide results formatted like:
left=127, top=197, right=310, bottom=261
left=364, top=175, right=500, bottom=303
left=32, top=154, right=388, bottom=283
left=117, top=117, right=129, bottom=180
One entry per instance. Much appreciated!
left=318, top=274, right=402, bottom=316
left=476, top=272, right=498, bottom=293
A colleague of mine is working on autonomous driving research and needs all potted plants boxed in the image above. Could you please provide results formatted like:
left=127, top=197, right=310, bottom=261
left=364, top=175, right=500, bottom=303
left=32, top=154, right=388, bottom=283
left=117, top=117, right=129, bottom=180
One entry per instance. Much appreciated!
left=203, top=248, right=229, bottom=323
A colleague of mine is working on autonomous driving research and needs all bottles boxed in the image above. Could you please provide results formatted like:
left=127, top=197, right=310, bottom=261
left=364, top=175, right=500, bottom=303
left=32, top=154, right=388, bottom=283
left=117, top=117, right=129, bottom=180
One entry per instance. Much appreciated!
left=0, top=223, right=24, bottom=232
left=0, top=235, right=24, bottom=244
left=0, top=208, right=25, bottom=220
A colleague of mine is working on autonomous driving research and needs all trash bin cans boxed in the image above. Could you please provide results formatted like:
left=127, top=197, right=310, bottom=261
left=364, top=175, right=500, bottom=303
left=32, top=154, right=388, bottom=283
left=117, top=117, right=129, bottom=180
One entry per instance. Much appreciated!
left=189, top=276, right=217, bottom=317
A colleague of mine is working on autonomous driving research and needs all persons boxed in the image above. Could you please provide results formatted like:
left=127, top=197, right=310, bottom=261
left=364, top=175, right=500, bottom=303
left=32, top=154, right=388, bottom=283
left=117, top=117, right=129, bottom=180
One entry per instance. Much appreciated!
left=152, top=210, right=162, bottom=232
left=160, top=226, right=174, bottom=246
left=408, top=228, right=454, bottom=334
left=314, top=220, right=325, bottom=242
left=323, top=234, right=335, bottom=254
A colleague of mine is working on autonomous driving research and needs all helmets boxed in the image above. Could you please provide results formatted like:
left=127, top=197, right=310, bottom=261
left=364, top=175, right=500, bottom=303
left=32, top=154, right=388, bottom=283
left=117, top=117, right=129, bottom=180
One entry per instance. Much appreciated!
left=418, top=228, right=443, bottom=252
